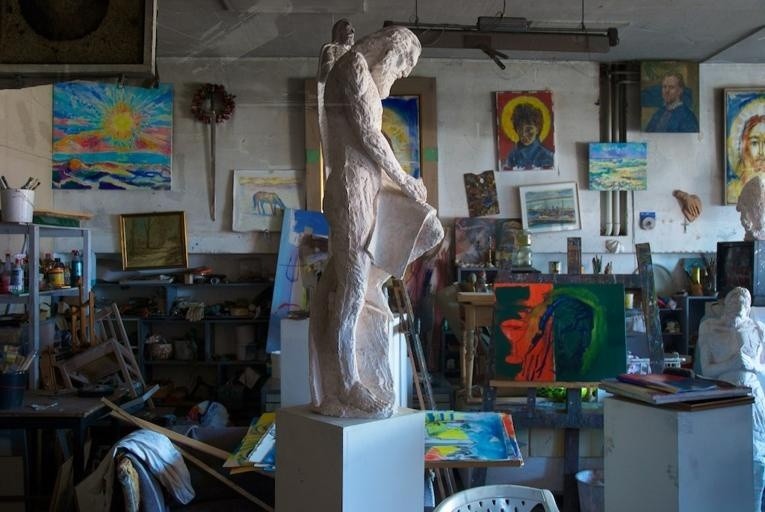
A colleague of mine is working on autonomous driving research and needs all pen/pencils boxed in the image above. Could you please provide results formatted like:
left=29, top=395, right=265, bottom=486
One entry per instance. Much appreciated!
left=12, top=350, right=37, bottom=374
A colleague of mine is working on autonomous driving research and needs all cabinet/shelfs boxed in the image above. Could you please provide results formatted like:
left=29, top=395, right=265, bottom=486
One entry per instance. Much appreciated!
left=95, top=281, right=274, bottom=408
left=455, top=271, right=724, bottom=401
left=0, top=220, right=94, bottom=406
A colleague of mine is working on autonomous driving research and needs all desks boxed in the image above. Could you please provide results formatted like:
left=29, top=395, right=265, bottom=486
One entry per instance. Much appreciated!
left=0, top=383, right=139, bottom=512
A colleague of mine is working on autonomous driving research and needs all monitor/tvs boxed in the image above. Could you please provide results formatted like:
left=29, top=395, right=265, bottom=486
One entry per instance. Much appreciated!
left=716, top=241, right=765, bottom=307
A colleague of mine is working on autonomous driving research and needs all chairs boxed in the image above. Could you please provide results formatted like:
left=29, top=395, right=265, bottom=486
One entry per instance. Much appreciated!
left=117, top=452, right=143, bottom=512
left=432, top=483, right=560, bottom=512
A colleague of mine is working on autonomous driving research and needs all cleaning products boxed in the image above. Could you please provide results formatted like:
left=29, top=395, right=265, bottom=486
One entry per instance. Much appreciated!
left=11, top=254, right=25, bottom=292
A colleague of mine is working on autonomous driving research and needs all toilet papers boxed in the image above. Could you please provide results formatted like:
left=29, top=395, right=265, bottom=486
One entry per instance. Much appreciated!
left=280, top=318, right=312, bottom=407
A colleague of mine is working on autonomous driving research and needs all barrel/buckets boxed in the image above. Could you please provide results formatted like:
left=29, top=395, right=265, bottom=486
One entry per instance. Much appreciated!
left=0, top=189, right=35, bottom=223
left=576, top=469, right=605, bottom=512
left=0, top=371, right=28, bottom=410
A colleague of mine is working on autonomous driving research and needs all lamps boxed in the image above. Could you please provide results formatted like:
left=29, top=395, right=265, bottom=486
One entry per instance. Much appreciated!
left=380, top=1, right=621, bottom=65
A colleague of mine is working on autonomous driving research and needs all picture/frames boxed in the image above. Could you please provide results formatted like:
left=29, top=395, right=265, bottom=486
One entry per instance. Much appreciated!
left=719, top=84, right=765, bottom=209
left=518, top=179, right=583, bottom=236
left=118, top=210, right=189, bottom=271
left=299, top=72, right=441, bottom=222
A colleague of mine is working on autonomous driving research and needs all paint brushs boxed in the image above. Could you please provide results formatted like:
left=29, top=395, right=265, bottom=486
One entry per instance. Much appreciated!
left=0, top=175, right=41, bottom=190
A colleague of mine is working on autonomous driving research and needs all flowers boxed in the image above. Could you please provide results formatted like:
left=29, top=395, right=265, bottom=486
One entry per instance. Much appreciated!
left=190, top=82, right=238, bottom=125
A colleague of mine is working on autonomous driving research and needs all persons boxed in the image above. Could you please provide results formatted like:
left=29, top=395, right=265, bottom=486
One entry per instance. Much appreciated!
left=320, top=18, right=356, bottom=82
left=506, top=102, right=553, bottom=169
left=645, top=72, right=698, bottom=133
left=726, top=114, right=765, bottom=202
left=699, top=286, right=765, bottom=512
left=306, top=24, right=428, bottom=412
